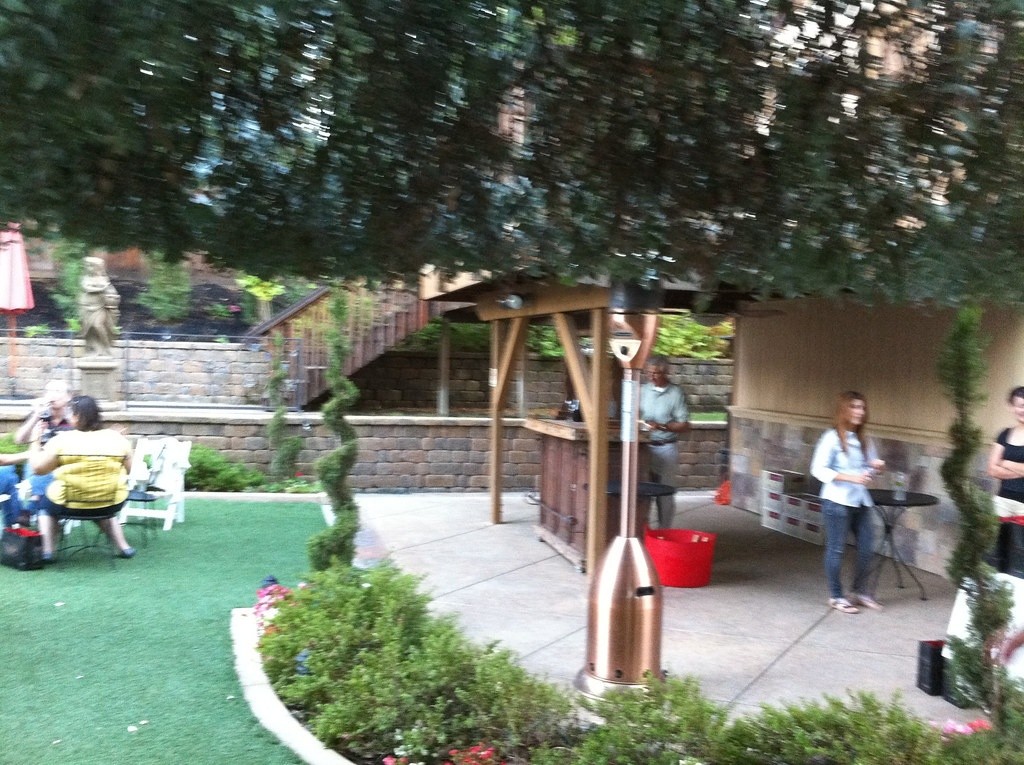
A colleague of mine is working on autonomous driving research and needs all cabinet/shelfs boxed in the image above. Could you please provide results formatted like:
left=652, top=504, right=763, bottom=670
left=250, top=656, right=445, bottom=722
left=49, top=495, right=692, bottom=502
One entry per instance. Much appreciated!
left=760, top=468, right=823, bottom=546
left=525, top=414, right=656, bottom=572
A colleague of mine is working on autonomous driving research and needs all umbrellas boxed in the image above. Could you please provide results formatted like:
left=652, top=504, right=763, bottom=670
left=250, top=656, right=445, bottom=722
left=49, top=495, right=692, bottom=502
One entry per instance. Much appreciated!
left=1, top=219, right=34, bottom=397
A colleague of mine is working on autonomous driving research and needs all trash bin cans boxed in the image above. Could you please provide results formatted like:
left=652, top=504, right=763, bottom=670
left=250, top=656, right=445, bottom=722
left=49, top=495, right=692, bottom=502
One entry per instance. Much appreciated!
left=642, top=523, right=717, bottom=589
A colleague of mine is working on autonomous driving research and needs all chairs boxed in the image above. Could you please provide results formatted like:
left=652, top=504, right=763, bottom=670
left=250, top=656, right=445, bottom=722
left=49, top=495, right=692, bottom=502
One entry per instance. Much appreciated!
left=53, top=455, right=128, bottom=561
left=117, top=436, right=192, bottom=531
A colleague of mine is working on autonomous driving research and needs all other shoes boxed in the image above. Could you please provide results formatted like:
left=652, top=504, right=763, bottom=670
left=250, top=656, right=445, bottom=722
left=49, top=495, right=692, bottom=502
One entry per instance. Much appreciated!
left=40, top=554, right=55, bottom=562
left=828, top=598, right=859, bottom=614
left=853, top=595, right=884, bottom=610
left=121, top=547, right=135, bottom=558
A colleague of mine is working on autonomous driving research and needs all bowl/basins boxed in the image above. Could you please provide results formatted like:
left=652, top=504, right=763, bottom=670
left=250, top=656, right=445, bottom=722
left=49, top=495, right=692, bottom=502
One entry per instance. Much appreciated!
left=644, top=523, right=717, bottom=587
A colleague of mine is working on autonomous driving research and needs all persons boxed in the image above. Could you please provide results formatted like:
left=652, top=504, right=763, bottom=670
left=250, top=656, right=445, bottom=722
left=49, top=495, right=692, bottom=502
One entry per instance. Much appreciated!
left=639, top=356, right=689, bottom=528
left=0, top=379, right=139, bottom=564
left=809, top=390, right=885, bottom=613
left=80, top=256, right=120, bottom=355
left=985, top=385, right=1024, bottom=577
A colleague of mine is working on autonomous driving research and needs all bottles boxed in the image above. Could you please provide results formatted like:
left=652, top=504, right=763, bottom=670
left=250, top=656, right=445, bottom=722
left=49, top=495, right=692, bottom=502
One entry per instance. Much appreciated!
left=855, top=463, right=878, bottom=490
left=895, top=473, right=907, bottom=500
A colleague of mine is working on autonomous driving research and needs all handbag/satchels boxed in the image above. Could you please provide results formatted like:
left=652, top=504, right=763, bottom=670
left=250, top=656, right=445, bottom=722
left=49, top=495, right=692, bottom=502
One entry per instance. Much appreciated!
left=0, top=527, right=44, bottom=571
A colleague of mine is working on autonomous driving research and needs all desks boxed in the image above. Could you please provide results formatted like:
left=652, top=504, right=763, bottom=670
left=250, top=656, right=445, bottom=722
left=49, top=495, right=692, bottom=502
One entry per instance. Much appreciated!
left=854, top=489, right=939, bottom=602
left=581, top=478, right=675, bottom=540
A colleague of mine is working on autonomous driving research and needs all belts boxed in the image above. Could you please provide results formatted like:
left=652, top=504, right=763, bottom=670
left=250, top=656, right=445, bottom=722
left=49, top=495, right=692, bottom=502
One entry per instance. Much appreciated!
left=650, top=440, right=676, bottom=446
